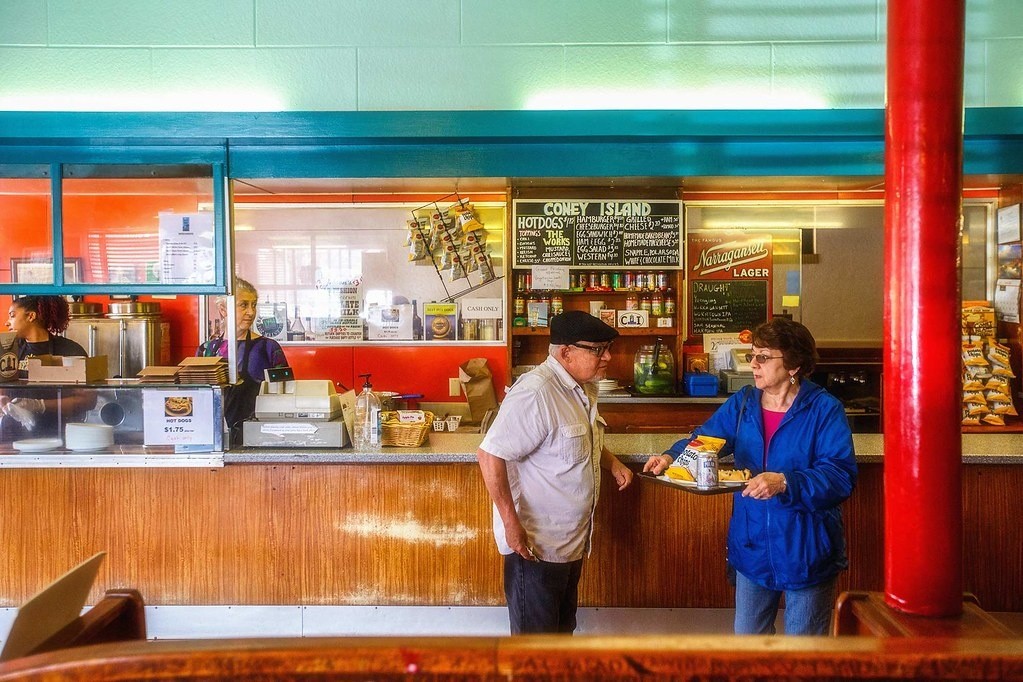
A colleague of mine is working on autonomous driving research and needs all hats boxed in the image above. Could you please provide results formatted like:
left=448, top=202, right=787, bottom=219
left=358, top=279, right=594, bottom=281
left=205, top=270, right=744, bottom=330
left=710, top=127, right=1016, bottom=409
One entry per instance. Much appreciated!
left=550, top=311, right=620, bottom=345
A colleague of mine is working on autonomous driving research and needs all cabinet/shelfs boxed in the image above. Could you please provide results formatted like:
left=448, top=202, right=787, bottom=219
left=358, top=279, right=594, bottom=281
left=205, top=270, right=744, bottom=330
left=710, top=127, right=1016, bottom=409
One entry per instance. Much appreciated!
left=504, top=185, right=689, bottom=398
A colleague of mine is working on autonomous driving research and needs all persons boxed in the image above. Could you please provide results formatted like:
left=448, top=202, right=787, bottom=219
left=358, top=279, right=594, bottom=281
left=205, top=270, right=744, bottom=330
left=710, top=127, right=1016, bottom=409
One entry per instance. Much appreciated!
left=478, top=309, right=634, bottom=637
left=196, top=276, right=293, bottom=450
left=0, top=295, right=97, bottom=445
left=643, top=316, right=858, bottom=637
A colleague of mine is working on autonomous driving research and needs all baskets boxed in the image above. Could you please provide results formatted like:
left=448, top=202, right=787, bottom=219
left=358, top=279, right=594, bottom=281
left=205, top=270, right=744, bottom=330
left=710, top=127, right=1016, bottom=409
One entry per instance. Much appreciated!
left=380, top=410, right=435, bottom=447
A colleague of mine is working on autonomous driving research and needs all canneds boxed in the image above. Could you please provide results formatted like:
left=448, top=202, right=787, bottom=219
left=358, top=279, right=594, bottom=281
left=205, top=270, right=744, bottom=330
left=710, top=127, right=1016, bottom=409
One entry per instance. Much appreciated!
left=697, top=449, right=719, bottom=489
left=513, top=270, right=669, bottom=290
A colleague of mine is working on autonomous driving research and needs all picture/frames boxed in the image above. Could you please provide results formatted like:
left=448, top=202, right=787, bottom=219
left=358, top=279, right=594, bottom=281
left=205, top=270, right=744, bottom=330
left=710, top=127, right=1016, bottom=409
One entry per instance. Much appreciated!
left=11, top=256, right=85, bottom=310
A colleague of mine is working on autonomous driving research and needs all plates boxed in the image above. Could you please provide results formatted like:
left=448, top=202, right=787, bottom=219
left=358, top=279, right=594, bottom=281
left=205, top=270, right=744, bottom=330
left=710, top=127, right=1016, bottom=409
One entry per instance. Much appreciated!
left=64, top=423, right=114, bottom=450
left=719, top=480, right=749, bottom=487
left=165, top=400, right=192, bottom=416
left=669, top=477, right=698, bottom=487
left=599, top=379, right=619, bottom=390
left=12, top=438, right=64, bottom=453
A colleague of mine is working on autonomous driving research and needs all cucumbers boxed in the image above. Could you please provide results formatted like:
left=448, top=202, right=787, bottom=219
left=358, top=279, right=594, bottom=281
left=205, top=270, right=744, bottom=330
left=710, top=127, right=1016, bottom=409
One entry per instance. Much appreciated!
left=633, top=362, right=674, bottom=394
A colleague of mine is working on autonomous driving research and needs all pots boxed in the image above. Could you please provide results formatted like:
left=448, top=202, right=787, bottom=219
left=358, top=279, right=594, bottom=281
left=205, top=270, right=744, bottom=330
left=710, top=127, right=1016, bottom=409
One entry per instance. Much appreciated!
left=356, top=391, right=424, bottom=410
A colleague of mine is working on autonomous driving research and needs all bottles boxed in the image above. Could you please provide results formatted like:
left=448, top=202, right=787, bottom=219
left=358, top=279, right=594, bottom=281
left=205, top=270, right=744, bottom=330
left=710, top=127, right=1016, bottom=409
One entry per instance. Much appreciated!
left=412, top=300, right=421, bottom=340
left=526, top=287, right=538, bottom=326
left=638, top=288, right=652, bottom=317
left=538, top=290, right=548, bottom=325
left=458, top=310, right=464, bottom=340
left=633, top=345, right=674, bottom=395
left=652, top=288, right=664, bottom=317
left=665, top=288, right=676, bottom=317
left=512, top=287, right=527, bottom=327
left=550, top=290, right=562, bottom=317
left=626, top=286, right=638, bottom=311
left=286, top=306, right=332, bottom=341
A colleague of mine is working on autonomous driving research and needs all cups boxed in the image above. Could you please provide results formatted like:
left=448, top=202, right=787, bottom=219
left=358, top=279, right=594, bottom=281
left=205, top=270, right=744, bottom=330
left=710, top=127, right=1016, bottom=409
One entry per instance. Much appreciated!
left=464, top=319, right=503, bottom=341
left=590, top=301, right=607, bottom=320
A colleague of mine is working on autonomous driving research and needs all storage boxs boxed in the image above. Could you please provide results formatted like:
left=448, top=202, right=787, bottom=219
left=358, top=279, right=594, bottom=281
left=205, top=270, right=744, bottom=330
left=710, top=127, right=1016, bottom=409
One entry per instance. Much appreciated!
left=683, top=371, right=719, bottom=397
left=720, top=371, right=757, bottom=394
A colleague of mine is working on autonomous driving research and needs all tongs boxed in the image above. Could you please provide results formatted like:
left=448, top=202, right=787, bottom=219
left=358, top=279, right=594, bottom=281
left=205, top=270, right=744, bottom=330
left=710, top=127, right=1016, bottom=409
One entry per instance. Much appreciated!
left=651, top=337, right=662, bottom=375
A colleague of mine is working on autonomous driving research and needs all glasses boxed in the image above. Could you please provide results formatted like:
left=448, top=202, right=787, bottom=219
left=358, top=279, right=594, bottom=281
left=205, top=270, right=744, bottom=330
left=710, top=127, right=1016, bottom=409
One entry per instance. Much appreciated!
left=572, top=341, right=613, bottom=356
left=745, top=354, right=783, bottom=363
